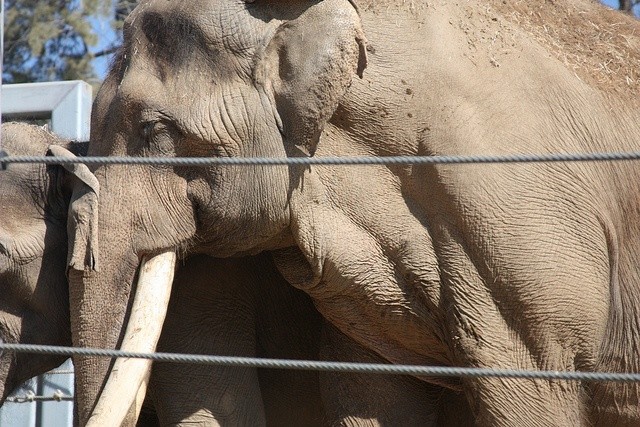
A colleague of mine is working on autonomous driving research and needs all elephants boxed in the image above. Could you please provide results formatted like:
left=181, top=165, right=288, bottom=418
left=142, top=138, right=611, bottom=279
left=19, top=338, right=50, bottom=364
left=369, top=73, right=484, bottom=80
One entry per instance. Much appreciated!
left=68, top=0, right=640, bottom=426
left=0, top=120, right=91, bottom=410
